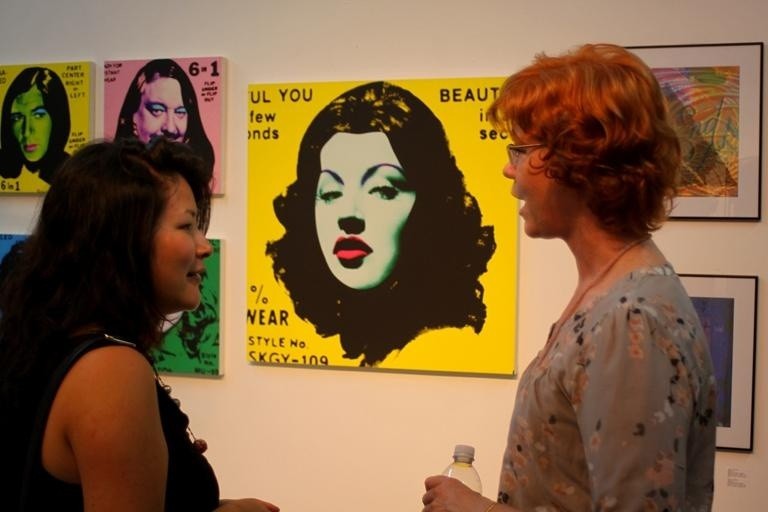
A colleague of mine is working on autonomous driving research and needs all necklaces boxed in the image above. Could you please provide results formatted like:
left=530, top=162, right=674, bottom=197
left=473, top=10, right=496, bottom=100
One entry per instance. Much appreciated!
left=537, top=233, right=653, bottom=356
left=154, top=372, right=209, bottom=455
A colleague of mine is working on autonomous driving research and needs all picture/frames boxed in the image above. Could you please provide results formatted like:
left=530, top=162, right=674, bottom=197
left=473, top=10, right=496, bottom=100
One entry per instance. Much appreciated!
left=618, top=42, right=762, bottom=221
left=676, top=274, right=758, bottom=451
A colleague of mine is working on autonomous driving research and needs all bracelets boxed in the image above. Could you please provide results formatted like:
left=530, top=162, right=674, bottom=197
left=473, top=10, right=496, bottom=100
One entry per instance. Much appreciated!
left=485, top=500, right=497, bottom=511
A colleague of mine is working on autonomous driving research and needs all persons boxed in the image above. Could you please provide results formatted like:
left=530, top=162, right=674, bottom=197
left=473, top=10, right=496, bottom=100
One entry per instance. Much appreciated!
left=0, top=137, right=283, bottom=511
left=111, top=60, right=215, bottom=183
left=0, top=68, right=72, bottom=184
left=264, top=82, right=498, bottom=367
left=419, top=41, right=718, bottom=511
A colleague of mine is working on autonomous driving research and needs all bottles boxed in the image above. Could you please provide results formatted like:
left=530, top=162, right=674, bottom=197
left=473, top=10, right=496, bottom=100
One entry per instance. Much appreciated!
left=442, top=445, right=482, bottom=496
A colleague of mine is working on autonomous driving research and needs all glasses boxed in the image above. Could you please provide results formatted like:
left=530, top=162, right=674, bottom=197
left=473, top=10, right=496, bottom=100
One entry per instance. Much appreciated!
left=505, top=141, right=542, bottom=165
